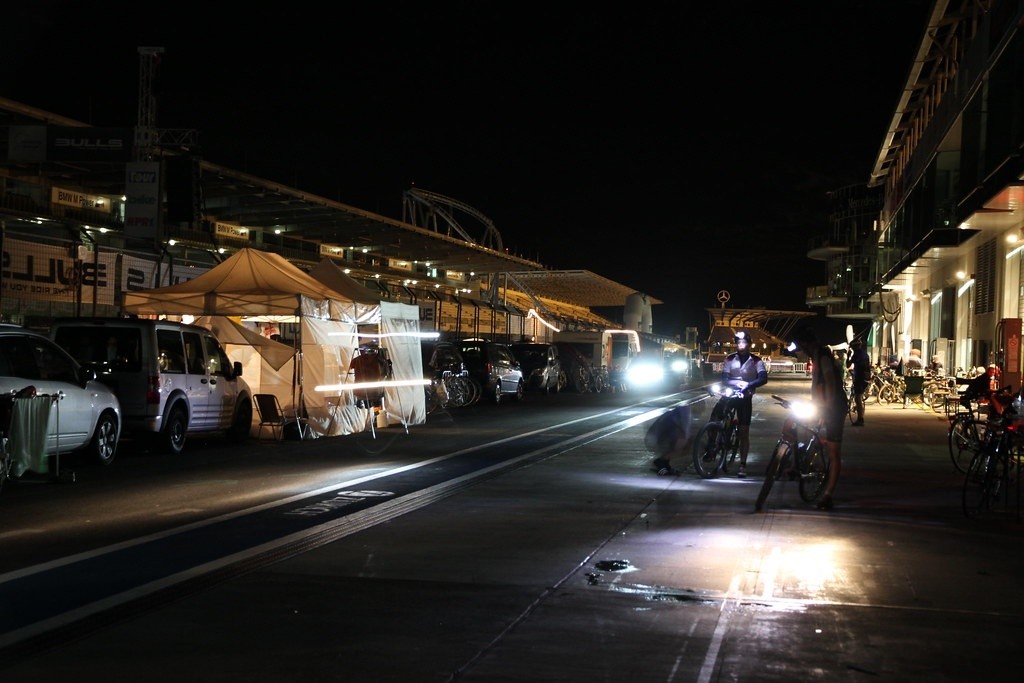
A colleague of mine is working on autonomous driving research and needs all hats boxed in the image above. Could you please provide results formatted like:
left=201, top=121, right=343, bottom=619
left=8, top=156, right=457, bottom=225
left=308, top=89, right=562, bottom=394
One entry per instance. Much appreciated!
left=735, top=330, right=750, bottom=343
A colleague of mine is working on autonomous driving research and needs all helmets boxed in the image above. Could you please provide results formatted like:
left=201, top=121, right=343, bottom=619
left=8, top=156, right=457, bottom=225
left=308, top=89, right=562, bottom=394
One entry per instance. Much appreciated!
left=848, top=339, right=862, bottom=348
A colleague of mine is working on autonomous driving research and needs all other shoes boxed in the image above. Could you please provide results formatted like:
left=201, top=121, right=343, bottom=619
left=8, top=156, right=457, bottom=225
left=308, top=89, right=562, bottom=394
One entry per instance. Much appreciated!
left=702, top=450, right=716, bottom=462
left=852, top=417, right=864, bottom=426
left=653, top=458, right=673, bottom=477
left=817, top=494, right=832, bottom=508
left=735, top=462, right=746, bottom=478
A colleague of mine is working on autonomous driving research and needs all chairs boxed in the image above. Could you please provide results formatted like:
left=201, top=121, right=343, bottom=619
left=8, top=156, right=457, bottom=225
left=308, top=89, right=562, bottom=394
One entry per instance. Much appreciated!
left=943, top=379, right=981, bottom=420
left=253, top=394, right=293, bottom=441
left=903, top=375, right=925, bottom=410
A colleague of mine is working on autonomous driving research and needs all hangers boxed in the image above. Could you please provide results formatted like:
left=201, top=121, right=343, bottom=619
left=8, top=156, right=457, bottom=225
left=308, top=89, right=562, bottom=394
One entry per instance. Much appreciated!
left=351, top=351, right=391, bottom=369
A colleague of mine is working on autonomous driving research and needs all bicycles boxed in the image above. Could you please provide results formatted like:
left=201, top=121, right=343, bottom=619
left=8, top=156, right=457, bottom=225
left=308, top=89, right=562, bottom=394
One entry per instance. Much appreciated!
left=836, top=362, right=1024, bottom=524
left=754, top=393, right=836, bottom=515
left=692, top=383, right=752, bottom=478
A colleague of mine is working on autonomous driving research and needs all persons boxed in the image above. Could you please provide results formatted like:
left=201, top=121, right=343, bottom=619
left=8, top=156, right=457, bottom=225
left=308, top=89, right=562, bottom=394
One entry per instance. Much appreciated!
left=701, top=331, right=768, bottom=476
left=644, top=398, right=706, bottom=477
left=797, top=327, right=849, bottom=509
left=846, top=339, right=872, bottom=427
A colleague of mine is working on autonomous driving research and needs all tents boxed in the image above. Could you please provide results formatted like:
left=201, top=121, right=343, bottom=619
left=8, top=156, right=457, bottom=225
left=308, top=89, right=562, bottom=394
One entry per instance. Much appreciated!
left=120, top=248, right=427, bottom=439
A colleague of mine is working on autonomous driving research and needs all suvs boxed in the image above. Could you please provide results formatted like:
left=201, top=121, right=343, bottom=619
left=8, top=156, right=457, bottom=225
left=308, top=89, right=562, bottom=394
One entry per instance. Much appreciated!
left=0, top=322, right=124, bottom=469
left=56, top=315, right=253, bottom=451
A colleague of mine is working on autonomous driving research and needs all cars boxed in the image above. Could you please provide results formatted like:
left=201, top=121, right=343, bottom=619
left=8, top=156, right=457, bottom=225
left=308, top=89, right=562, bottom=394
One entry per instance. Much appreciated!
left=359, top=328, right=692, bottom=420
